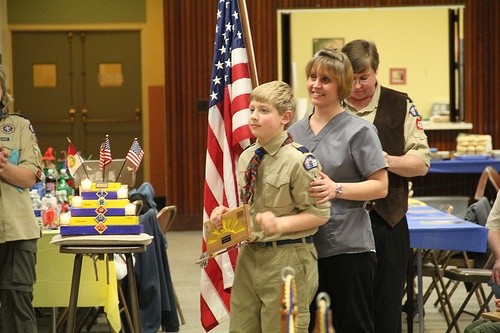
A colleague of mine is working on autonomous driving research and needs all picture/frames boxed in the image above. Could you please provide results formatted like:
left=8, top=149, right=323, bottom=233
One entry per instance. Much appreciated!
left=390, top=68, right=407, bottom=85
left=313, top=37, right=344, bottom=58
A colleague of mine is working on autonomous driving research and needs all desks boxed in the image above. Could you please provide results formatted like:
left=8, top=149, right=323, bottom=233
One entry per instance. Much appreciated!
left=408, top=155, right=500, bottom=197
left=58, top=245, right=146, bottom=333
left=31, top=226, right=122, bottom=333
left=405, top=198, right=489, bottom=333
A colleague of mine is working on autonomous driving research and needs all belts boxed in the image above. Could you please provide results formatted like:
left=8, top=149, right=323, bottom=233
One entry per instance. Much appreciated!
left=248, top=236, right=313, bottom=248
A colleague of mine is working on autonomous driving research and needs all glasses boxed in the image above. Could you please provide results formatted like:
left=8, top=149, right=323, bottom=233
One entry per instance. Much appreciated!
left=351, top=71, right=376, bottom=86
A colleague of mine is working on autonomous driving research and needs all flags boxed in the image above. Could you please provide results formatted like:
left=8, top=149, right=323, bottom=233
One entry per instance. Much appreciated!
left=114, top=138, right=144, bottom=182
left=66, top=136, right=90, bottom=179
left=199, top=0, right=259, bottom=333
left=99, top=134, right=112, bottom=182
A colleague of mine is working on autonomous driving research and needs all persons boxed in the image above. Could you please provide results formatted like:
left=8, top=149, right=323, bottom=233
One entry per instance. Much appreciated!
left=285, top=48, right=388, bottom=333
left=0, top=66, right=43, bottom=333
left=211, top=81, right=331, bottom=333
left=485, top=189, right=500, bottom=299
left=464, top=299, right=500, bottom=333
left=339, top=40, right=431, bottom=333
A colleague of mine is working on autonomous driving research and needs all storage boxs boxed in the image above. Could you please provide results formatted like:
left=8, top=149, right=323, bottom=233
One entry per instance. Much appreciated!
left=204, top=204, right=253, bottom=254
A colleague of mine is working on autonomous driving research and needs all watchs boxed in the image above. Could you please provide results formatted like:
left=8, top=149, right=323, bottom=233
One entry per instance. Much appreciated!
left=335, top=181, right=343, bottom=199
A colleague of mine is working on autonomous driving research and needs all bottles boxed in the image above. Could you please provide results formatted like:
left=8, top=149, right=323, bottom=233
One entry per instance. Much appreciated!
left=29, top=168, right=71, bottom=230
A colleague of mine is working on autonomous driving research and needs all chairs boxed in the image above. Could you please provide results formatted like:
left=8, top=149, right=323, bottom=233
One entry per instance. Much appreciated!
left=51, top=183, right=187, bottom=333
left=406, top=198, right=500, bottom=333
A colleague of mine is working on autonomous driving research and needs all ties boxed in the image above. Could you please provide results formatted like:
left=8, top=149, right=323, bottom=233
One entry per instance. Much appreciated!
left=240, top=133, right=293, bottom=204
